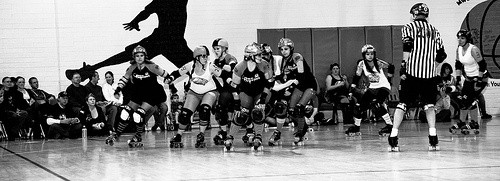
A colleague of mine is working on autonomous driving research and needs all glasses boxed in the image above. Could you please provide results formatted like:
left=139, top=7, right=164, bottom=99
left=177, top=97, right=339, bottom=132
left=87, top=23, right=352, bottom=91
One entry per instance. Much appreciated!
left=5, top=81, right=11, bottom=83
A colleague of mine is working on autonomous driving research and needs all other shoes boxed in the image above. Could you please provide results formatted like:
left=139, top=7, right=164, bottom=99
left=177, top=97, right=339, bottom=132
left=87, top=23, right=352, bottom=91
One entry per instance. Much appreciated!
left=481, top=114, right=492, bottom=119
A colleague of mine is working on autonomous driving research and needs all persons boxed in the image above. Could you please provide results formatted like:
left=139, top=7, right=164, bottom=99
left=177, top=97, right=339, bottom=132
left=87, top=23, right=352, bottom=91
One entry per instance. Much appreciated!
left=0, top=3, right=492, bottom=151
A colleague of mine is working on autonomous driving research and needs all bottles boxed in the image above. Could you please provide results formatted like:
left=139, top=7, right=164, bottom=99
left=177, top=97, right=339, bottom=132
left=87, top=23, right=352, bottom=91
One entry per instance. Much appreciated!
left=81, top=125, right=87, bottom=139
left=81, top=139, right=87, bottom=152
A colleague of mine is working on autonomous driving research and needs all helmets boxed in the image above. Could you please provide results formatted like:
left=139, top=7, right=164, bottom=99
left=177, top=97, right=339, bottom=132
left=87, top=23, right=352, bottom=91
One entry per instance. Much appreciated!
left=278, top=38, right=295, bottom=50
left=361, top=44, right=376, bottom=53
left=410, top=3, right=429, bottom=18
left=211, top=38, right=228, bottom=50
left=193, top=46, right=210, bottom=58
left=244, top=42, right=264, bottom=56
left=457, top=29, right=471, bottom=42
left=260, top=44, right=273, bottom=54
left=132, top=45, right=147, bottom=59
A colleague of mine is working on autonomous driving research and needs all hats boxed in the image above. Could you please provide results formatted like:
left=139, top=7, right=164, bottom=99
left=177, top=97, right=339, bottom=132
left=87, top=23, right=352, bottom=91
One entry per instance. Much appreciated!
left=58, top=91, right=70, bottom=99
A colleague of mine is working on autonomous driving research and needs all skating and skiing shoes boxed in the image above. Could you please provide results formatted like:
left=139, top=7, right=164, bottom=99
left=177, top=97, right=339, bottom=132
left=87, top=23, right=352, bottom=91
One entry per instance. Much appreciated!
left=345, top=124, right=362, bottom=136
left=449, top=119, right=467, bottom=134
left=378, top=123, right=393, bottom=136
left=128, top=135, right=143, bottom=148
left=389, top=134, right=400, bottom=152
left=106, top=127, right=121, bottom=147
left=428, top=135, right=440, bottom=151
left=171, top=125, right=313, bottom=151
left=461, top=120, right=479, bottom=135
